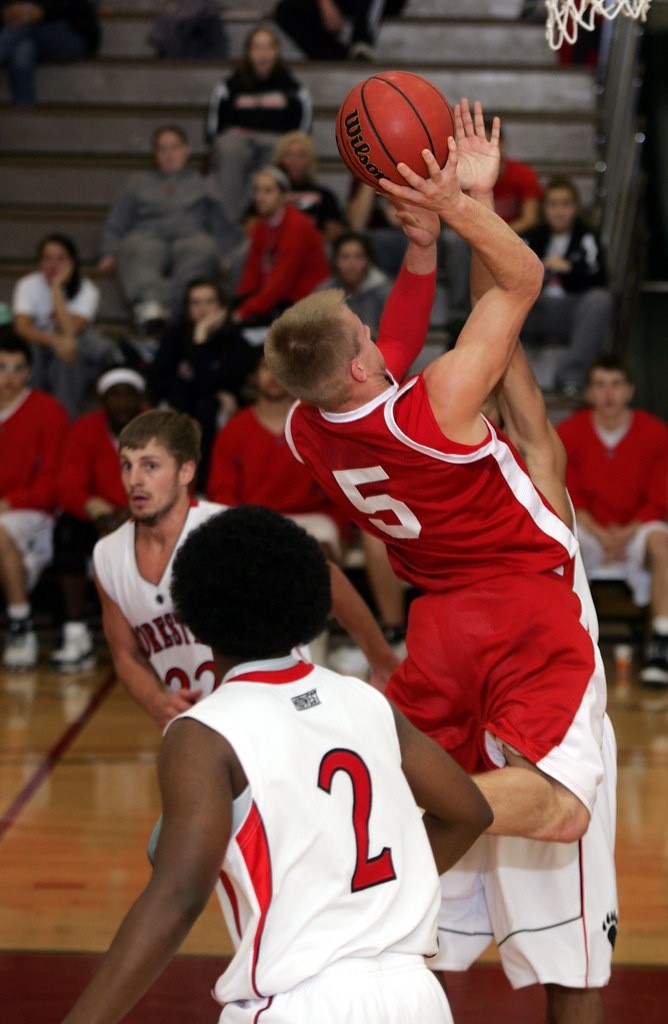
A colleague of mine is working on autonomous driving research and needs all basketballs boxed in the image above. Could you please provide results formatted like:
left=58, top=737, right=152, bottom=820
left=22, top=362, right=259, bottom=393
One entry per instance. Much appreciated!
left=334, top=70, right=456, bottom=195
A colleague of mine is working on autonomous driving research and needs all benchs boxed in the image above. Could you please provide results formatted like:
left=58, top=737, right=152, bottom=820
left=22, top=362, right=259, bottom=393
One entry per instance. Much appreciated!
left=3, top=0, right=666, bottom=684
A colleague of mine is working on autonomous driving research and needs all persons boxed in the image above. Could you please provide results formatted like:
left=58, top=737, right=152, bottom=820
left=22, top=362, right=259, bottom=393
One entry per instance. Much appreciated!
left=419, top=97, right=619, bottom=1023
left=0, top=0, right=668, bottom=687
left=63, top=505, right=494, bottom=1024
left=93, top=410, right=402, bottom=865
left=265, top=135, right=605, bottom=841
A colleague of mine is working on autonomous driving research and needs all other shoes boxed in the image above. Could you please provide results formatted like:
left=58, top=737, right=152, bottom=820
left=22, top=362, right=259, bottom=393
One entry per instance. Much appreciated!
left=137, top=301, right=164, bottom=329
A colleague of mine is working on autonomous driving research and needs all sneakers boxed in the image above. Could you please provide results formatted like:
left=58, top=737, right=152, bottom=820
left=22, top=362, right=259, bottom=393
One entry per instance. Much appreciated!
left=640, top=629, right=668, bottom=684
left=51, top=624, right=95, bottom=671
left=5, top=612, right=39, bottom=668
